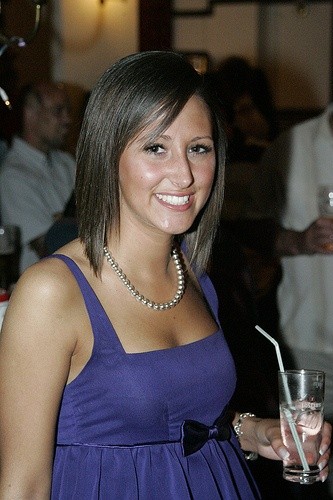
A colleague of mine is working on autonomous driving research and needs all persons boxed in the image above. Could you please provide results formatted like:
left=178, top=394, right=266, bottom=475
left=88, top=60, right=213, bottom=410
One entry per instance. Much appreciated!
left=1, top=82, right=76, bottom=277
left=249, top=105, right=333, bottom=421
left=0, top=49, right=333, bottom=500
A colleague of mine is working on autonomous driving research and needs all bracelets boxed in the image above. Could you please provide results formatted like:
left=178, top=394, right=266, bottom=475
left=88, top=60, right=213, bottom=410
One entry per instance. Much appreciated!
left=229, top=402, right=262, bottom=462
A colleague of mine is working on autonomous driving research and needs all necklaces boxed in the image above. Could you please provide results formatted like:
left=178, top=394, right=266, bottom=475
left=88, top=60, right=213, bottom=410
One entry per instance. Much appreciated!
left=92, top=229, right=200, bottom=322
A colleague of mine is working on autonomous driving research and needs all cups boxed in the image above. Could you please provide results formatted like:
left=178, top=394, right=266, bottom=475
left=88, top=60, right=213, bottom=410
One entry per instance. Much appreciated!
left=316, top=184, right=333, bottom=252
left=277, top=368, right=325, bottom=484
